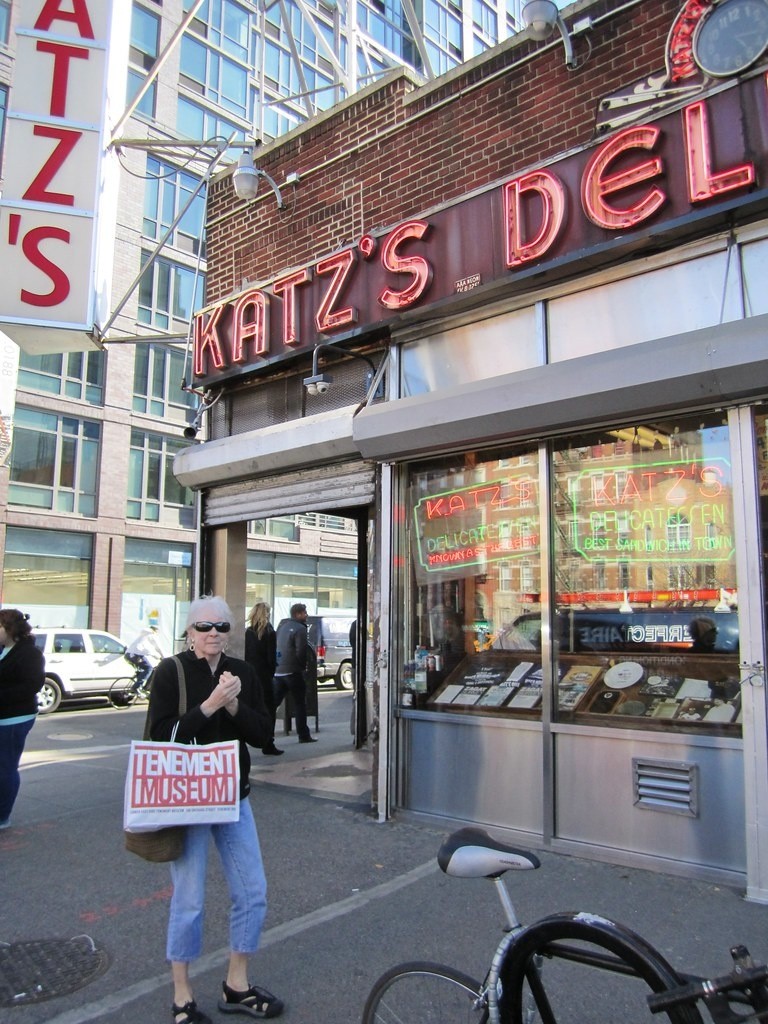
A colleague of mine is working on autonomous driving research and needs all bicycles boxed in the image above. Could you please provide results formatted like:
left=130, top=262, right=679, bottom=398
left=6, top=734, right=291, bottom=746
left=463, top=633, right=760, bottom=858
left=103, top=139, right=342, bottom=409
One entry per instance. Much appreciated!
left=107, top=655, right=160, bottom=711
left=360, top=827, right=768, bottom=1023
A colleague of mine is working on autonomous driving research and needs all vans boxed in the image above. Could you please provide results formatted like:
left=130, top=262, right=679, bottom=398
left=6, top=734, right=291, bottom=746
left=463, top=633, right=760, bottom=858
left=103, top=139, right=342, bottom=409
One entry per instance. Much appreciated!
left=280, top=616, right=354, bottom=691
left=493, top=588, right=741, bottom=653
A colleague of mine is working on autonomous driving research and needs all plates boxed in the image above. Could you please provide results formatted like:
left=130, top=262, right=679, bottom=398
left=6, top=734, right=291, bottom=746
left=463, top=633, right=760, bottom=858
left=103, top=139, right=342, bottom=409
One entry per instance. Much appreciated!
left=603, top=662, right=643, bottom=688
left=618, top=701, right=645, bottom=715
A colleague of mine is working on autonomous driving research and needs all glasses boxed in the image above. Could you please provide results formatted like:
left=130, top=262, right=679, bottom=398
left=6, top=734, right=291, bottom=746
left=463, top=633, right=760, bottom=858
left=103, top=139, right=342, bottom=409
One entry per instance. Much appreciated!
left=191, top=621, right=230, bottom=633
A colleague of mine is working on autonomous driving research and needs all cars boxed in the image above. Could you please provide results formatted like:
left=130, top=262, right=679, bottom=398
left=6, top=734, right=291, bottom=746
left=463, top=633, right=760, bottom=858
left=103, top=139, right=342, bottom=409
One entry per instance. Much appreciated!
left=26, top=627, right=163, bottom=715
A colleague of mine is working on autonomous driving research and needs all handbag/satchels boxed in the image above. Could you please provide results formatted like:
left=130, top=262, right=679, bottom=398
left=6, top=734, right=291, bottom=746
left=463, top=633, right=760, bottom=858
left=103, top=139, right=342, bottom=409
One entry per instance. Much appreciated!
left=125, top=828, right=184, bottom=861
left=123, top=721, right=239, bottom=832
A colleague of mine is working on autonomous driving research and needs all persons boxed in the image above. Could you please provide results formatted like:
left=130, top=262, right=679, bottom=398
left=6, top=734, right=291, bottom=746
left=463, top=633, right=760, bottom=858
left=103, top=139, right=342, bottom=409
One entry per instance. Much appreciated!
left=275, top=604, right=319, bottom=743
left=125, top=617, right=167, bottom=700
left=245, top=602, right=284, bottom=756
left=348, top=620, right=357, bottom=745
left=142, top=596, right=284, bottom=1024
left=688, top=617, right=730, bottom=655
left=0, top=609, right=46, bottom=828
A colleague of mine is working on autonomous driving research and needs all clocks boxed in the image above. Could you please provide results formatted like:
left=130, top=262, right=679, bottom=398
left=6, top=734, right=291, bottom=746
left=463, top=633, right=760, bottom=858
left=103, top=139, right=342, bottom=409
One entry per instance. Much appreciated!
left=690, top=0, right=768, bottom=77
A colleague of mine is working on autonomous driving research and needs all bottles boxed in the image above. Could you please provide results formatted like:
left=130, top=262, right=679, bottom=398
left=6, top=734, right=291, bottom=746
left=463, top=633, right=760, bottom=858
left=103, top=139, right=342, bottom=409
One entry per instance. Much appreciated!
left=400, top=646, right=441, bottom=709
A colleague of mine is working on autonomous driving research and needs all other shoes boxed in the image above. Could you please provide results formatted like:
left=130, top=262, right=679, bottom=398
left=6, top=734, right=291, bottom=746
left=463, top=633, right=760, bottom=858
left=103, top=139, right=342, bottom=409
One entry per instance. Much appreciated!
left=132, top=686, right=141, bottom=694
left=0, top=821, right=11, bottom=829
left=262, top=746, right=285, bottom=755
left=300, top=737, right=318, bottom=743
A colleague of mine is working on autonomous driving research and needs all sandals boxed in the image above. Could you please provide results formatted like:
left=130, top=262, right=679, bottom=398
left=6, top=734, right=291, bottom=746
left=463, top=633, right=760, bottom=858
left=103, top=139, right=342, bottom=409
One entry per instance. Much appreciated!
left=219, top=980, right=284, bottom=1017
left=173, top=999, right=212, bottom=1024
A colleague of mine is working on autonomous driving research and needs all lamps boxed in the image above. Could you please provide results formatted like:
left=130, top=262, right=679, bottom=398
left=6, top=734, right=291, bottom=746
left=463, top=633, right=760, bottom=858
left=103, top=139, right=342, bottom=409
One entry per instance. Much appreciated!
left=523, top=1, right=593, bottom=72
left=184, top=385, right=227, bottom=439
left=305, top=343, right=387, bottom=400
left=233, top=148, right=299, bottom=224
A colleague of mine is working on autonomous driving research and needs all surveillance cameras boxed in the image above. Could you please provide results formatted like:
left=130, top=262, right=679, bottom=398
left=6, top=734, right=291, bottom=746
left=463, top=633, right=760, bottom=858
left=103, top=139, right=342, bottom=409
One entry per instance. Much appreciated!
left=306, top=382, right=330, bottom=395
